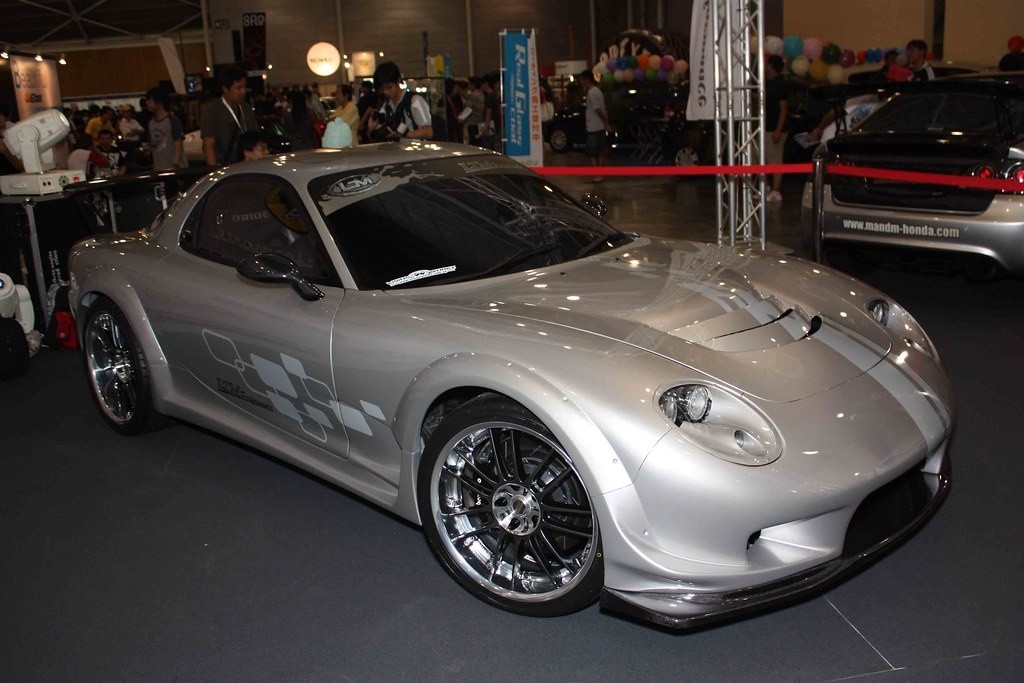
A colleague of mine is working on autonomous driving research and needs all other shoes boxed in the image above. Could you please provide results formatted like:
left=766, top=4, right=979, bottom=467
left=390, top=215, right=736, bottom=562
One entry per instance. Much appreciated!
left=584, top=178, right=603, bottom=184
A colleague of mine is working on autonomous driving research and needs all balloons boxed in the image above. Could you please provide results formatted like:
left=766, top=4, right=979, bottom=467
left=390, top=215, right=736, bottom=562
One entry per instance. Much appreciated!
left=751, top=35, right=906, bottom=85
left=598, top=55, right=688, bottom=84
left=1007, top=36, right=1024, bottom=52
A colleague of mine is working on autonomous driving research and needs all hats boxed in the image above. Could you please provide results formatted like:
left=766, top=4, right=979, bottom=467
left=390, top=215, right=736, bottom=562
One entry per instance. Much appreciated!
left=121, top=105, right=132, bottom=112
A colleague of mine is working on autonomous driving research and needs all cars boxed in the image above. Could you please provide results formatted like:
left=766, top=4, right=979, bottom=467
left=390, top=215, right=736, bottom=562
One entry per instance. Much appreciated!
left=800, top=69, right=1024, bottom=285
left=542, top=87, right=715, bottom=180
left=319, top=96, right=336, bottom=112
left=181, top=114, right=292, bottom=164
left=63, top=138, right=958, bottom=631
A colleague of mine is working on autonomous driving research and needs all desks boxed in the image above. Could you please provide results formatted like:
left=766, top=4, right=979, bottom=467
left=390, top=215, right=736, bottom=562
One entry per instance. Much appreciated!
left=0, top=164, right=222, bottom=327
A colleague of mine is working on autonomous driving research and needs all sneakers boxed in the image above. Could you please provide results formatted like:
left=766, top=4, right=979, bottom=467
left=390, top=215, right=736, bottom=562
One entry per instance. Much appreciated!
left=753, top=187, right=782, bottom=203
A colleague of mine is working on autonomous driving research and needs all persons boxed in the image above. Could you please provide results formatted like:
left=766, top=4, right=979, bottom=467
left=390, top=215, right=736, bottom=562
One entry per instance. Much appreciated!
left=567, top=69, right=612, bottom=183
left=752, top=55, right=790, bottom=202
left=877, top=39, right=935, bottom=100
left=441, top=69, right=558, bottom=167
left=0, top=61, right=434, bottom=236
left=781, top=88, right=848, bottom=226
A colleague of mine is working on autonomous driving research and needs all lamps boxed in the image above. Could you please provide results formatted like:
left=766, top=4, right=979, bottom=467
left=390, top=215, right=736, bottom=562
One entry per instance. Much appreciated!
left=35, top=49, right=43, bottom=61
left=0, top=46, right=10, bottom=59
left=0, top=108, right=87, bottom=196
left=59, top=53, right=66, bottom=65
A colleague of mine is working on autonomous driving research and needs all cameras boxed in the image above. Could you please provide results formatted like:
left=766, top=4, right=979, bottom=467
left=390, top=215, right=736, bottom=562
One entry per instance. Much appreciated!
left=371, top=112, right=397, bottom=140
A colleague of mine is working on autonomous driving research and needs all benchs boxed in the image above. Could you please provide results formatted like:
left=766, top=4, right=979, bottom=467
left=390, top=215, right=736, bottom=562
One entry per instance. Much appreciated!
left=194, top=183, right=447, bottom=273
